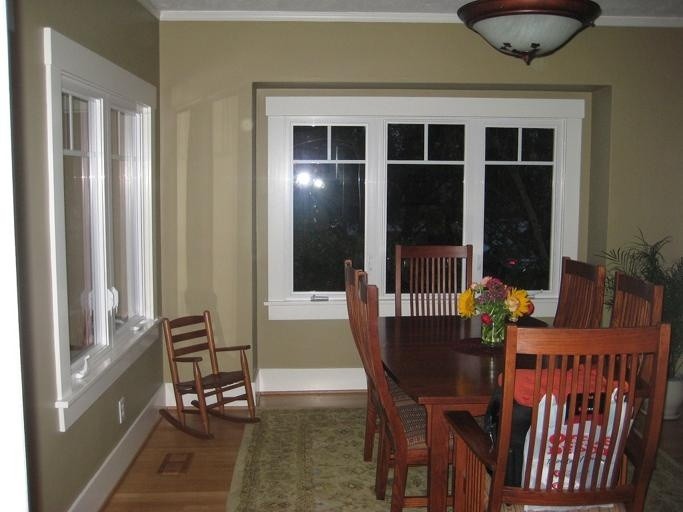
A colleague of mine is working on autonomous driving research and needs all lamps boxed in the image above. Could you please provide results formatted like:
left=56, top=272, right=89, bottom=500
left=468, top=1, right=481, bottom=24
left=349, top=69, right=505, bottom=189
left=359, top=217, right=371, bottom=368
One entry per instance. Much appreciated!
left=451, top=1, right=601, bottom=69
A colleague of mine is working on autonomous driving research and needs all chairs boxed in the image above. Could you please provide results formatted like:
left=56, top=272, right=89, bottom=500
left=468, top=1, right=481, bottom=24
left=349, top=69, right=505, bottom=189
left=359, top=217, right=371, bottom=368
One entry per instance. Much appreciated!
left=394, top=243, right=474, bottom=319
left=603, top=271, right=666, bottom=435
left=162, top=310, right=263, bottom=441
left=441, top=319, right=672, bottom=510
left=356, top=271, right=483, bottom=511
left=553, top=255, right=606, bottom=328
left=342, top=257, right=417, bottom=502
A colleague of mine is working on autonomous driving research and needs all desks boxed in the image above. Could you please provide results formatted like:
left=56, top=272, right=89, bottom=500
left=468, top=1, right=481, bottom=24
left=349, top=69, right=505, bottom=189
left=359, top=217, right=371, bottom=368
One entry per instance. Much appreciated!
left=379, top=316, right=654, bottom=511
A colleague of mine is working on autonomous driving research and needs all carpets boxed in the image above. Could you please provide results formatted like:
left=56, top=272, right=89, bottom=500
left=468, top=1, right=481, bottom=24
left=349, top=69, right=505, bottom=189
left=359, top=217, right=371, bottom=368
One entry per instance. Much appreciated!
left=226, top=403, right=682, bottom=512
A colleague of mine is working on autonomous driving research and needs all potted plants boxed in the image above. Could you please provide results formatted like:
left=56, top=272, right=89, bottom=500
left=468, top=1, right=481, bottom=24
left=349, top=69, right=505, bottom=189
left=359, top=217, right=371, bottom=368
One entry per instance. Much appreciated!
left=590, top=216, right=681, bottom=422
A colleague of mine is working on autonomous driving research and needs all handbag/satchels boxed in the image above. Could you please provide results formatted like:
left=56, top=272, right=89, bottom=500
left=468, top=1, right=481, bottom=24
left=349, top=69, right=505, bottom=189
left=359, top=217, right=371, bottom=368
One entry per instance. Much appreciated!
left=484, top=365, right=613, bottom=505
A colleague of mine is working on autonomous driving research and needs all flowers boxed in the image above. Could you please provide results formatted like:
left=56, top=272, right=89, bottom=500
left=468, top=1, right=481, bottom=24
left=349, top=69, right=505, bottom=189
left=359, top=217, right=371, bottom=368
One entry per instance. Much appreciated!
left=453, top=275, right=532, bottom=341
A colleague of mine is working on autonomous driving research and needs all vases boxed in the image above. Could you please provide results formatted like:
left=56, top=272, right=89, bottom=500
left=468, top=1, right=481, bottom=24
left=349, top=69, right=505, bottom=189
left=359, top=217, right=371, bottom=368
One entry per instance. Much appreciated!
left=478, top=311, right=505, bottom=342
